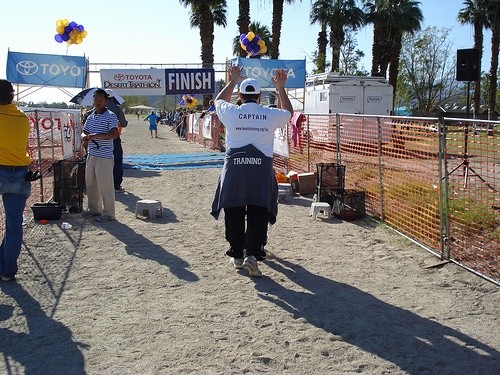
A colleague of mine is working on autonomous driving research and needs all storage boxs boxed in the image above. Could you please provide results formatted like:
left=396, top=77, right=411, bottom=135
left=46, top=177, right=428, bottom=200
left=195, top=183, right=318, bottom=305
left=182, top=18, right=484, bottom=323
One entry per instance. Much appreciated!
left=298, top=163, right=366, bottom=222
left=31, top=159, right=85, bottom=222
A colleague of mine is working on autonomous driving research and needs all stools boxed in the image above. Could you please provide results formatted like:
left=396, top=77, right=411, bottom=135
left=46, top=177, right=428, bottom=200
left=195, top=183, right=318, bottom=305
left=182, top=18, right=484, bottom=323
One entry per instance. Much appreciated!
left=135, top=200, right=162, bottom=220
left=310, top=202, right=331, bottom=220
left=278, top=183, right=294, bottom=202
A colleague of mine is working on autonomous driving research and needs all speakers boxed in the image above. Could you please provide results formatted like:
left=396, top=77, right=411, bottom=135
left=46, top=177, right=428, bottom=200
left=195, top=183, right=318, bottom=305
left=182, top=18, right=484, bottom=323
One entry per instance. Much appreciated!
left=457, top=49, right=481, bottom=82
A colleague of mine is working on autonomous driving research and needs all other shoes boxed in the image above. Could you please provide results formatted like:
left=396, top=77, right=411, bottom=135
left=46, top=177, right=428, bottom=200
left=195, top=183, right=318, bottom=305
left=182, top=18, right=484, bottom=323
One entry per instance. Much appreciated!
left=150, top=136, right=153, bottom=138
left=0, top=275, right=14, bottom=281
left=115, top=186, right=125, bottom=193
left=256, top=249, right=266, bottom=261
left=155, top=136, right=158, bottom=138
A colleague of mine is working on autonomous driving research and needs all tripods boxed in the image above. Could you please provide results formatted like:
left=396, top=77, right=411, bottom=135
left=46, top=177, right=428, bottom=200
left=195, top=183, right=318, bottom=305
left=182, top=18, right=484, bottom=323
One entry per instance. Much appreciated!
left=442, top=82, right=498, bottom=193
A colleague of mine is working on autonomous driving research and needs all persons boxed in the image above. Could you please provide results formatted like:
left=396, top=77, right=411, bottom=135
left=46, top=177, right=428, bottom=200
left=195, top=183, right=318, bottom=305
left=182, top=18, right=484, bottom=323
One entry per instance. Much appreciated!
left=0, top=79, right=30, bottom=280
left=81, top=64, right=294, bottom=277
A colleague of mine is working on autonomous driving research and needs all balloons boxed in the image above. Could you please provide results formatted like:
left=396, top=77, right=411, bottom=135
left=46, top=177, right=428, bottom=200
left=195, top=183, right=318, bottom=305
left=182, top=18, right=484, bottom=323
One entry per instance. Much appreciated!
left=240, top=31, right=267, bottom=59
left=55, top=18, right=86, bottom=45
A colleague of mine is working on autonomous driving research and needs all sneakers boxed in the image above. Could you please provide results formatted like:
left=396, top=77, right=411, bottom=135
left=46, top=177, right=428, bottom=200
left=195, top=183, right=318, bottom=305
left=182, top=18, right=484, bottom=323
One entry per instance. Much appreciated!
left=95, top=214, right=115, bottom=222
left=230, top=257, right=244, bottom=269
left=242, top=256, right=262, bottom=277
left=80, top=210, right=102, bottom=218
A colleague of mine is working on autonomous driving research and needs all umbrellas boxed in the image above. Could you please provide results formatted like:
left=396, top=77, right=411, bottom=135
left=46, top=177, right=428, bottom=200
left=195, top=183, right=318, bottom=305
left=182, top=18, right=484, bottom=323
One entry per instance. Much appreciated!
left=70, top=85, right=125, bottom=107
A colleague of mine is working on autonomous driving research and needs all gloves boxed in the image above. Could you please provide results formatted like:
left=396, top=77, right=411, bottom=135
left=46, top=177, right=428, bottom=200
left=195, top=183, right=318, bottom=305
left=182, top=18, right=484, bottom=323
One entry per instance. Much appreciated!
left=25, top=169, right=43, bottom=182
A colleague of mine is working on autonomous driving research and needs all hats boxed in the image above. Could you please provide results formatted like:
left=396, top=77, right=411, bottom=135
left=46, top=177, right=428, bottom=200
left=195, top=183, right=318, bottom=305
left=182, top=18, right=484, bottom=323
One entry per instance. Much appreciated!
left=104, top=88, right=114, bottom=98
left=0, top=78, right=15, bottom=97
left=238, top=78, right=261, bottom=95
